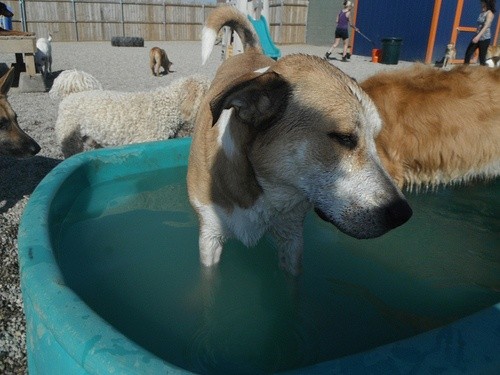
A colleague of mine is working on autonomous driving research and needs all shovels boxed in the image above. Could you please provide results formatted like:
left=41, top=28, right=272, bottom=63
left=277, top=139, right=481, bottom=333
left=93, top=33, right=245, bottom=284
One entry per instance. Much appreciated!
left=349, top=23, right=376, bottom=47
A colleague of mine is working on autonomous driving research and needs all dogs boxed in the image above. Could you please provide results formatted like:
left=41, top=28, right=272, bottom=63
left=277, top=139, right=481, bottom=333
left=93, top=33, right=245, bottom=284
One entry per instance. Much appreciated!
left=358, top=62, right=500, bottom=194
left=474, top=45, right=500, bottom=64
left=148, top=45, right=175, bottom=79
left=0, top=63, right=43, bottom=162
left=439, top=40, right=457, bottom=69
left=35, top=32, right=54, bottom=80
left=184, top=1, right=415, bottom=326
left=48, top=69, right=209, bottom=160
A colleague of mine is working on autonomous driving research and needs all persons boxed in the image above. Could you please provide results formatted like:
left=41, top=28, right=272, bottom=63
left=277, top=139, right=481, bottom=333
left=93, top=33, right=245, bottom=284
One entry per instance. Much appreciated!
left=325, top=0, right=354, bottom=61
left=465, top=0, right=495, bottom=66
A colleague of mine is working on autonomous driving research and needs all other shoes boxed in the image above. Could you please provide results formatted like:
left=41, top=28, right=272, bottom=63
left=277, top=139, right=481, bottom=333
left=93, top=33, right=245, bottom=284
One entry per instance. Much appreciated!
left=326, top=52, right=332, bottom=59
left=342, top=56, right=347, bottom=61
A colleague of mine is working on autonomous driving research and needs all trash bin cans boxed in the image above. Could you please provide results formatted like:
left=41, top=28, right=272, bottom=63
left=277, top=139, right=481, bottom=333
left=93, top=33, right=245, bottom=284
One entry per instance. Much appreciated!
left=373, top=49, right=381, bottom=62
left=381, top=37, right=402, bottom=64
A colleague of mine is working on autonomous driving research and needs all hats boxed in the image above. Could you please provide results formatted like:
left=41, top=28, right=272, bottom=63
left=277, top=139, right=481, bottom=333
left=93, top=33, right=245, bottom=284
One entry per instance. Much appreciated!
left=343, top=0, right=354, bottom=7
